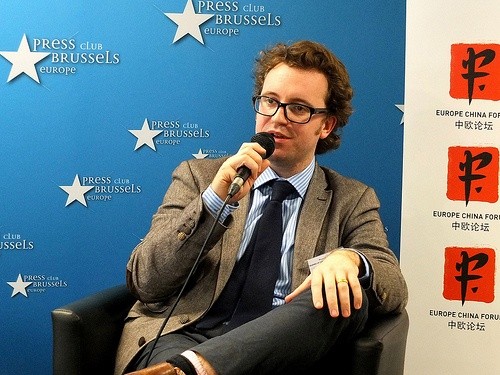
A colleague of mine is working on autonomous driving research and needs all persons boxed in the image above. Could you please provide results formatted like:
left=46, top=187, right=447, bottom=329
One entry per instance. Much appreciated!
left=114, top=41, right=408, bottom=375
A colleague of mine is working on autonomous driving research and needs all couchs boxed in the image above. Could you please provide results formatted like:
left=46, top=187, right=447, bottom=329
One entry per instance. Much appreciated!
left=51, top=284, right=409, bottom=375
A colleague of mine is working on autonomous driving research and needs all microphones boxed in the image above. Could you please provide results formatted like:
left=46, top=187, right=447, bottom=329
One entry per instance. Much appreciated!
left=227, top=132, right=275, bottom=197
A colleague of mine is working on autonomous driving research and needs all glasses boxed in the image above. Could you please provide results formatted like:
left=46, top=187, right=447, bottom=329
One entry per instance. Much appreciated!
left=252, top=95, right=330, bottom=124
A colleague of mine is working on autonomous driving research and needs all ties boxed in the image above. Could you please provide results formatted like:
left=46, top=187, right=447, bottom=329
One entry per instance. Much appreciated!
left=196, top=180, right=301, bottom=328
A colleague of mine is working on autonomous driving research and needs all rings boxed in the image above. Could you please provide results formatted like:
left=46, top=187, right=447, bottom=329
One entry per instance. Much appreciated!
left=337, top=279, right=348, bottom=284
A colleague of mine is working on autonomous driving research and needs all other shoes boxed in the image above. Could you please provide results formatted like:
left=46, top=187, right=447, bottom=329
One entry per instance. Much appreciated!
left=124, top=362, right=186, bottom=375
